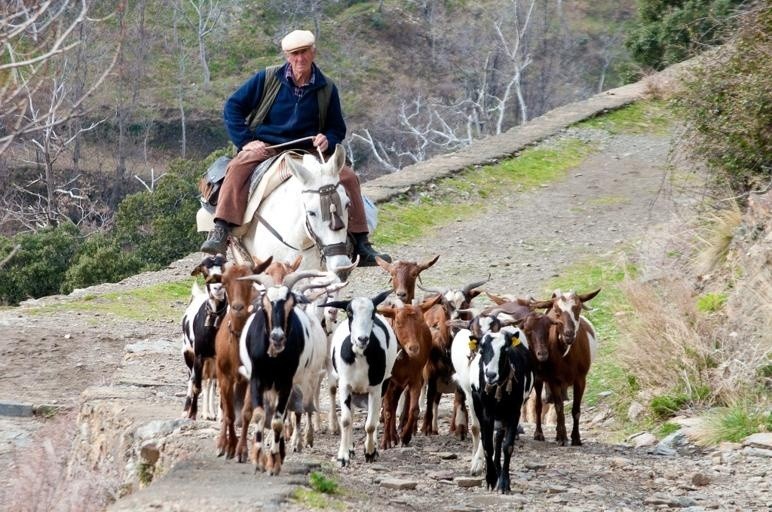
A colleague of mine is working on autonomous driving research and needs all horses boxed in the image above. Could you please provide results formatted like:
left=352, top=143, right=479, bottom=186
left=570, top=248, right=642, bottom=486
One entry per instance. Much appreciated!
left=197, top=142, right=355, bottom=283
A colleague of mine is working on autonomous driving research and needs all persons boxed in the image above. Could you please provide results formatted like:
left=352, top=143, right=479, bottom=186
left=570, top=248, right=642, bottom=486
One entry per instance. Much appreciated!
left=198, top=26, right=392, bottom=272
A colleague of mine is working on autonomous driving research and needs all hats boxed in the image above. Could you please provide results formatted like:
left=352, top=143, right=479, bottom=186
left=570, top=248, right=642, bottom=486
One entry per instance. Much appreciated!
left=282, top=30, right=315, bottom=53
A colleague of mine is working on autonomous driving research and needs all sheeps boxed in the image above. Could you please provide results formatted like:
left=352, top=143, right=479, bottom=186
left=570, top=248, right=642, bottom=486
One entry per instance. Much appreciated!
left=181, top=254, right=602, bottom=496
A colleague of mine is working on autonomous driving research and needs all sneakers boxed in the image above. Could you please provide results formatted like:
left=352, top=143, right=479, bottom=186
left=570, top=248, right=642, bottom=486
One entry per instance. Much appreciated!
left=352, top=242, right=392, bottom=267
left=201, top=224, right=229, bottom=257
